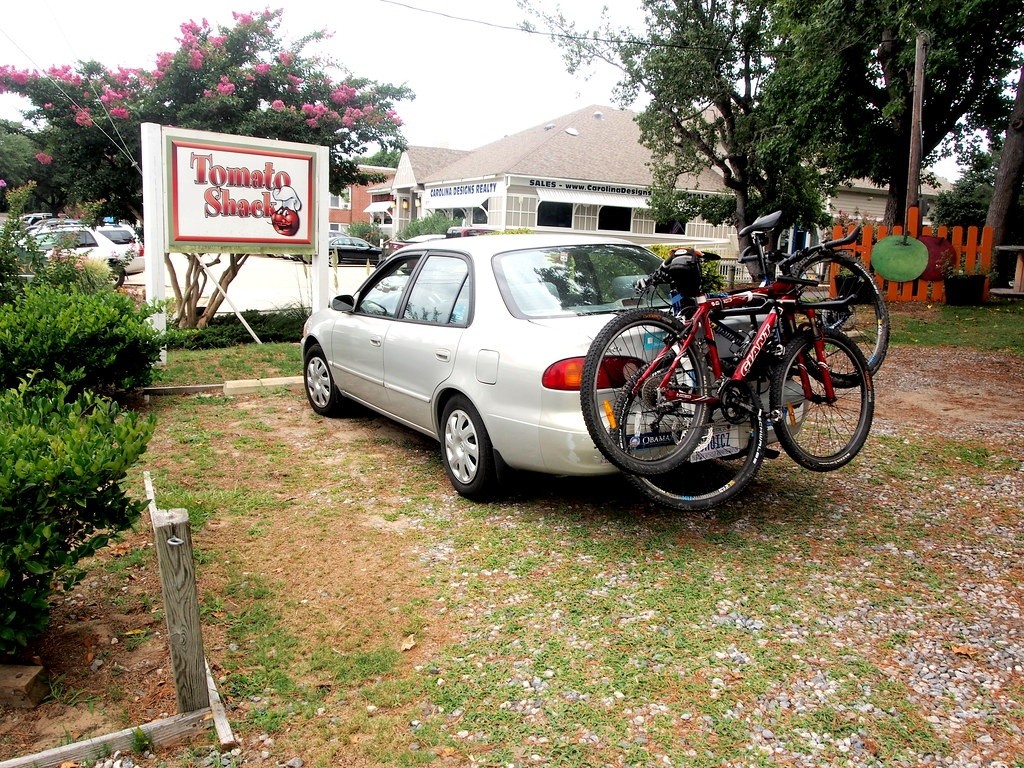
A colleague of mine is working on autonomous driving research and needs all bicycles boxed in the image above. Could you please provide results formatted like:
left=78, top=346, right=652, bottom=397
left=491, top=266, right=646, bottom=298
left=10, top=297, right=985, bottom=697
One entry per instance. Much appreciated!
left=580, top=206, right=892, bottom=513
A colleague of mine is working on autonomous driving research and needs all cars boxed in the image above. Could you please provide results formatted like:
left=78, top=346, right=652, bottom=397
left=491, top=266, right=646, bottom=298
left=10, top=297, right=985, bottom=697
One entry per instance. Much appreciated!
left=387, top=234, right=446, bottom=277
left=299, top=232, right=810, bottom=503
left=0, top=211, right=146, bottom=290
left=329, top=232, right=352, bottom=241
left=328, top=235, right=386, bottom=268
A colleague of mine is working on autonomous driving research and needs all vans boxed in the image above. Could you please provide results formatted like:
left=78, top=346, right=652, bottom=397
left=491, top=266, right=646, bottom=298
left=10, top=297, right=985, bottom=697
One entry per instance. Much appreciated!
left=446, top=227, right=498, bottom=239
